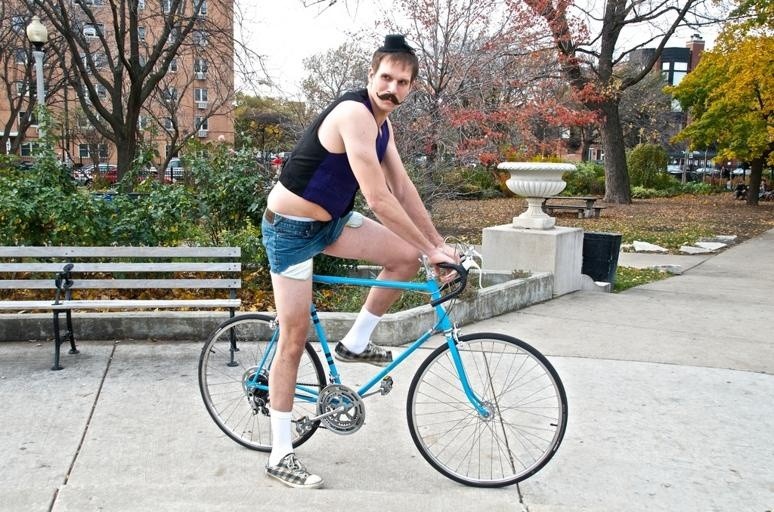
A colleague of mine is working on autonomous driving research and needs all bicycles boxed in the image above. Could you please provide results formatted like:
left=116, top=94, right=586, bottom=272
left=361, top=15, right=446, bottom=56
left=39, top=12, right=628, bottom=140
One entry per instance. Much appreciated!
left=198, top=235, right=567, bottom=488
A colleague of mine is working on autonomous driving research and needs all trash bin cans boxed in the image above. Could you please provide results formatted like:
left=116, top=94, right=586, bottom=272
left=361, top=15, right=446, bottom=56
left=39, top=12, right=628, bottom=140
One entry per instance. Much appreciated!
left=581, top=232, right=622, bottom=293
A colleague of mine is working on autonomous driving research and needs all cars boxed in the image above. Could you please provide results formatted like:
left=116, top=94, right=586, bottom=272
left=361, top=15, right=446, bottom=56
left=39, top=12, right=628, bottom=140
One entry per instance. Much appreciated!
left=667, top=164, right=752, bottom=185
left=252, top=151, right=293, bottom=168
left=70, top=159, right=187, bottom=188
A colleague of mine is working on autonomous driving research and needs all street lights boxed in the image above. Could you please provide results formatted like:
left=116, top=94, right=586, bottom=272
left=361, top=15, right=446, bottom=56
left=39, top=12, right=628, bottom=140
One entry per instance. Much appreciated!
left=25, top=13, right=49, bottom=162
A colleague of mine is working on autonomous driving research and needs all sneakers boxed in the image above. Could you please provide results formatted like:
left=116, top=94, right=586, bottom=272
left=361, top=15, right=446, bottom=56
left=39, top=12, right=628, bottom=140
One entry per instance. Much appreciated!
left=264, top=452, right=322, bottom=489
left=335, top=341, right=402, bottom=363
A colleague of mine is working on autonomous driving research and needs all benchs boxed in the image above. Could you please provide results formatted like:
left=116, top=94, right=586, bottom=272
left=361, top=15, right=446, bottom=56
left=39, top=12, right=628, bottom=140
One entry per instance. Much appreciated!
left=521, top=204, right=607, bottom=219
left=0, top=247, right=241, bottom=370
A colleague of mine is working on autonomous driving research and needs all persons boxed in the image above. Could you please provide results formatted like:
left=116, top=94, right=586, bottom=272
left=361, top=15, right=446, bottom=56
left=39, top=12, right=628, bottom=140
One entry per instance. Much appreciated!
left=271, top=155, right=282, bottom=173
left=759, top=179, right=767, bottom=199
left=259, top=34, right=462, bottom=491
left=735, top=180, right=747, bottom=200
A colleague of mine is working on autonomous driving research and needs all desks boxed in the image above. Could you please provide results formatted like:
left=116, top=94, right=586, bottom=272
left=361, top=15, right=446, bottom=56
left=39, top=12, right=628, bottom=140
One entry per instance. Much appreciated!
left=546, top=196, right=598, bottom=211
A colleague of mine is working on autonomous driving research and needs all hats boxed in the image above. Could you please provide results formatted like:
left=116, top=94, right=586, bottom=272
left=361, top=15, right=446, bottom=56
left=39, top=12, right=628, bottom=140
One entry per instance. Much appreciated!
left=379, top=34, right=412, bottom=53
